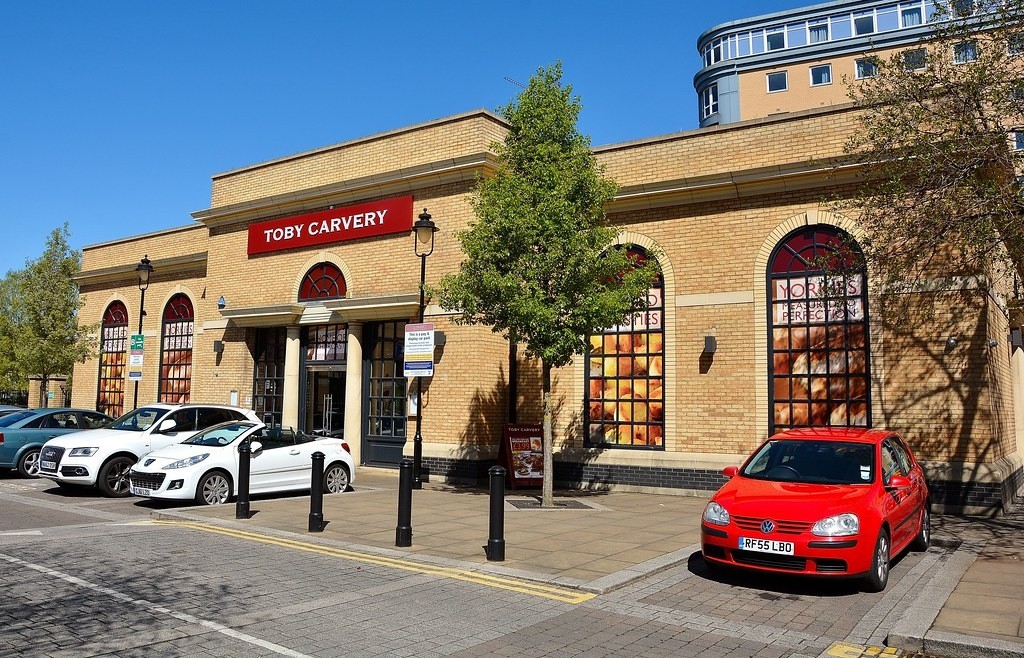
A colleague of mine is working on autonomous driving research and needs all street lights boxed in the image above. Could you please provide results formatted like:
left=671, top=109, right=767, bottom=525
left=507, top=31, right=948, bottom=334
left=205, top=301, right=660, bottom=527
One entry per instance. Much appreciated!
left=412, top=207, right=440, bottom=490
left=133, top=254, right=154, bottom=410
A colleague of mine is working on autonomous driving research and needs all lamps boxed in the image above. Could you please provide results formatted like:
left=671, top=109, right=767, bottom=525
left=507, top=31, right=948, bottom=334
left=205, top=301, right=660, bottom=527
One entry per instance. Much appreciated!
left=704, top=334, right=717, bottom=353
left=214, top=340, right=224, bottom=353
left=433, top=330, right=445, bottom=347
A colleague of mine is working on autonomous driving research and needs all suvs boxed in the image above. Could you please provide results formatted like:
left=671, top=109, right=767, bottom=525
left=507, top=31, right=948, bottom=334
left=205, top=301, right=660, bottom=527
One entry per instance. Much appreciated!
left=37, top=403, right=262, bottom=498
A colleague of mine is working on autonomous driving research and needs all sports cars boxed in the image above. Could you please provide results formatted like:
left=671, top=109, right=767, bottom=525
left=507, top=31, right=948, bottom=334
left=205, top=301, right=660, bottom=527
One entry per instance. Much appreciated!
left=130, top=420, right=356, bottom=505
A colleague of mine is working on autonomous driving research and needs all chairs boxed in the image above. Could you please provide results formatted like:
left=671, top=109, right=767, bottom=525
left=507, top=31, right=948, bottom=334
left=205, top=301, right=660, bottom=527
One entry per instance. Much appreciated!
left=64, top=419, right=77, bottom=428
left=48, top=417, right=60, bottom=428
left=793, top=444, right=819, bottom=467
left=245, top=429, right=263, bottom=449
left=260, top=427, right=284, bottom=451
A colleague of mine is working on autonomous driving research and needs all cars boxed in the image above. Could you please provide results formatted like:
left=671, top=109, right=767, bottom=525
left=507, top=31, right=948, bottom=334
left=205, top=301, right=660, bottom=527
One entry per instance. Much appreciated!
left=700, top=427, right=932, bottom=593
left=0, top=405, right=115, bottom=479
